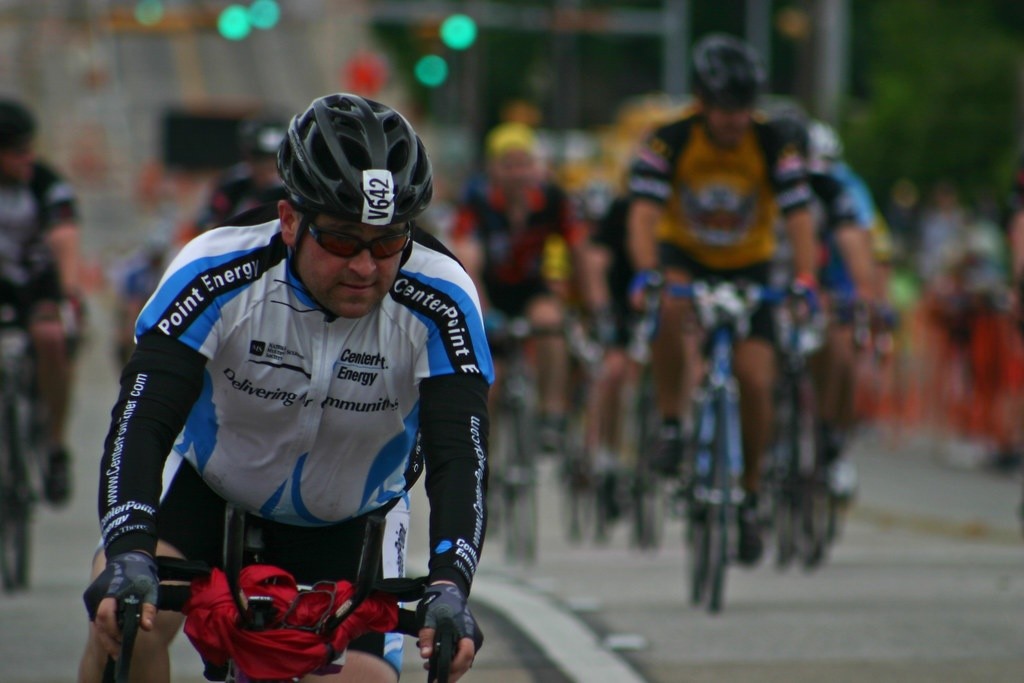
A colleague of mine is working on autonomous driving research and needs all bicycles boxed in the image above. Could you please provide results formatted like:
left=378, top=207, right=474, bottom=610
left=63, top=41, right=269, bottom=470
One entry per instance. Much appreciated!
left=475, top=257, right=885, bottom=614
left=0, top=295, right=78, bottom=593
left=101, top=498, right=455, bottom=683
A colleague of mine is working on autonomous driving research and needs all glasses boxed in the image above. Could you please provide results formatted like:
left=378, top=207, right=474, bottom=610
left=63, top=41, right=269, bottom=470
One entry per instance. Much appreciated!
left=308, top=220, right=411, bottom=259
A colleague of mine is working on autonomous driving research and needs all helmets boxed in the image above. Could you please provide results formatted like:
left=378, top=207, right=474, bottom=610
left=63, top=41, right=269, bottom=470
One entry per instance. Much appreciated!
left=485, top=121, right=540, bottom=158
left=686, top=35, right=758, bottom=105
left=0, top=100, right=36, bottom=148
left=808, top=119, right=844, bottom=159
left=276, top=93, right=433, bottom=225
left=239, top=107, right=290, bottom=157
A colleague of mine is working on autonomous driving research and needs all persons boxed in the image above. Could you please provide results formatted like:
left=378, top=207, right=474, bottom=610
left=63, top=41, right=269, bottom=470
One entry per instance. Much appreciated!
left=79, top=94, right=496, bottom=683
left=0, top=34, right=1024, bottom=566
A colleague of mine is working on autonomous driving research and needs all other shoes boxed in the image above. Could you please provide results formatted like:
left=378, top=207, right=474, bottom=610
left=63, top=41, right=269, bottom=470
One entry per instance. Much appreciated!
left=43, top=446, right=71, bottom=507
